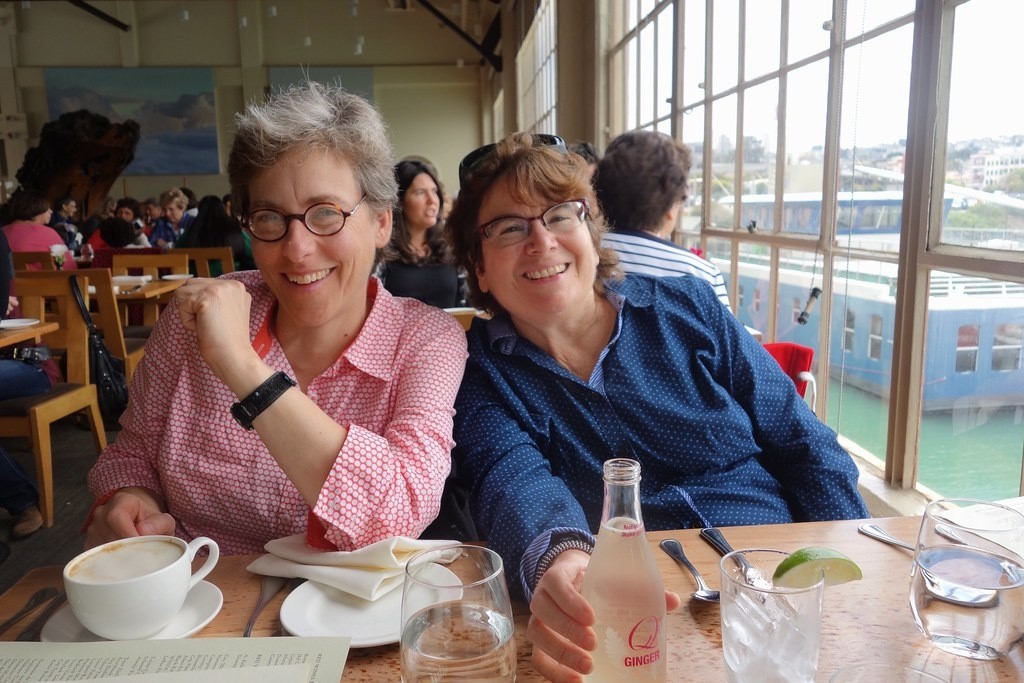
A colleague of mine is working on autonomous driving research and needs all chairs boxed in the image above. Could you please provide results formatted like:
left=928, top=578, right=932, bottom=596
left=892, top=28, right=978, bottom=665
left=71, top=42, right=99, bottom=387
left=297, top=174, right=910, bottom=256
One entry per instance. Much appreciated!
left=9, top=245, right=234, bottom=528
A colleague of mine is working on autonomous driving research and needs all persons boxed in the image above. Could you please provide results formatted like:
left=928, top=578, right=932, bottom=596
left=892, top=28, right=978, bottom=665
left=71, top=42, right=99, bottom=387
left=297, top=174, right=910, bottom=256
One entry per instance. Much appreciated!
left=0, top=229, right=59, bottom=562
left=449, top=133, right=872, bottom=682
left=84, top=84, right=469, bottom=550
left=2, top=186, right=257, bottom=277
left=591, top=130, right=735, bottom=318
left=374, top=160, right=469, bottom=308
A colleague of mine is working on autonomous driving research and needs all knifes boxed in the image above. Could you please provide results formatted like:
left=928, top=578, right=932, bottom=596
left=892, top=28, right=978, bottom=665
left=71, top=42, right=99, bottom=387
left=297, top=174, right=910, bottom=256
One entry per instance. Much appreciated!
left=15, top=591, right=67, bottom=642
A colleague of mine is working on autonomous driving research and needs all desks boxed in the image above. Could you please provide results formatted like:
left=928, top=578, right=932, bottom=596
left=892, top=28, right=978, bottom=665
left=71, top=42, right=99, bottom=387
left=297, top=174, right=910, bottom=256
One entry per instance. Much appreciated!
left=89, top=280, right=187, bottom=299
left=0, top=514, right=1024, bottom=683
left=0, top=320, right=59, bottom=348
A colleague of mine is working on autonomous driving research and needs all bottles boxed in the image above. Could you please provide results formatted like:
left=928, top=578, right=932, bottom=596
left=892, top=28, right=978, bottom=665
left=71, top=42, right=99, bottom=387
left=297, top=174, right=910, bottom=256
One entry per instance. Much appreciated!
left=581, top=457, right=667, bottom=683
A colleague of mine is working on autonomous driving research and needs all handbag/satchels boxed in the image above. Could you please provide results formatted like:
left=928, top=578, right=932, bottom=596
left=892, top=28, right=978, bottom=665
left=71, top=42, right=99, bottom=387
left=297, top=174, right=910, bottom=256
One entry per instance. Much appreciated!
left=2, top=344, right=60, bottom=389
left=69, top=275, right=129, bottom=418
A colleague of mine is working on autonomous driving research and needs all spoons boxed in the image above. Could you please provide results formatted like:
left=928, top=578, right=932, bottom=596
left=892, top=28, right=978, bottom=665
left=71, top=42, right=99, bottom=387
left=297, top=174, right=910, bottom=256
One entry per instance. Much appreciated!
left=658, top=539, right=721, bottom=604
left=242, top=577, right=284, bottom=637
left=858, top=521, right=957, bottom=573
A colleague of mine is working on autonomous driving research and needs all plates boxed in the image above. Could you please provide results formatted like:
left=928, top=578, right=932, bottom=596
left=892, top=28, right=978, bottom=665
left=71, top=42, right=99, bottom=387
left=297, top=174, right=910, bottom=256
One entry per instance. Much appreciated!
left=87, top=274, right=194, bottom=293
left=40, top=581, right=223, bottom=642
left=279, top=560, right=464, bottom=648
left=0, top=318, right=39, bottom=329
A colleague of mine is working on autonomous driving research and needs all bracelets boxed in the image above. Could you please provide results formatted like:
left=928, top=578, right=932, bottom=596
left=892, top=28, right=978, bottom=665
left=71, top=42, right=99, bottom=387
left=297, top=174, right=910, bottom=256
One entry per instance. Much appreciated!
left=534, top=540, right=594, bottom=584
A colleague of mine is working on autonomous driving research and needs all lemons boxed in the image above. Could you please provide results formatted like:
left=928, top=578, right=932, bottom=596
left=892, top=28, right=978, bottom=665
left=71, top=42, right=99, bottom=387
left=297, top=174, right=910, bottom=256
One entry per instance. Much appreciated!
left=772, top=547, right=862, bottom=589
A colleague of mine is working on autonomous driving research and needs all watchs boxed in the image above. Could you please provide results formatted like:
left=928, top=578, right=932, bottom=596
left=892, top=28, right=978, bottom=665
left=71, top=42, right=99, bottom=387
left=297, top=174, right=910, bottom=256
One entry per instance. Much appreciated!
left=231, top=371, right=297, bottom=432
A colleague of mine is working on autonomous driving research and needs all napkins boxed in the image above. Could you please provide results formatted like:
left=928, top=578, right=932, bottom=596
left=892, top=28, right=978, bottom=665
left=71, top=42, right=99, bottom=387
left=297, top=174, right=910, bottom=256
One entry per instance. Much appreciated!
left=245, top=532, right=463, bottom=601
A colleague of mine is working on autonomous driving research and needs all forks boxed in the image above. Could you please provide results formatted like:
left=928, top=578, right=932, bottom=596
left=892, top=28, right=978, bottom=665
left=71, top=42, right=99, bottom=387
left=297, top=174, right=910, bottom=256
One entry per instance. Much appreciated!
left=934, top=524, right=1022, bottom=584
left=701, top=528, right=798, bottom=621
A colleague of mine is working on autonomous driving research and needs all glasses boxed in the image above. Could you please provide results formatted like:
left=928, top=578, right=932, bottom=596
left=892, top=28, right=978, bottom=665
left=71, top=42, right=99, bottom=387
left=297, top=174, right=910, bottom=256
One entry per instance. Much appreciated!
left=459, top=134, right=569, bottom=188
left=241, top=193, right=367, bottom=242
left=479, top=198, right=590, bottom=249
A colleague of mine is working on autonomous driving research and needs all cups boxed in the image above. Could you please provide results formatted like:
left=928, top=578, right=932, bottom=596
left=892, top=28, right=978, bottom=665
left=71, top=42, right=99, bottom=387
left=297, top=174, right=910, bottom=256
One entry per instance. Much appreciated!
left=401, top=544, right=516, bottom=683
left=80, top=244, right=92, bottom=259
left=908, top=497, right=1023, bottom=660
left=720, top=548, right=825, bottom=683
left=63, top=534, right=220, bottom=641
